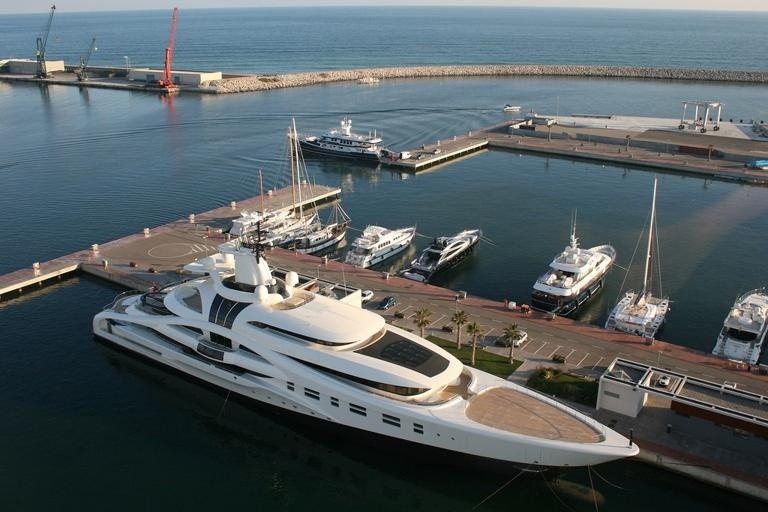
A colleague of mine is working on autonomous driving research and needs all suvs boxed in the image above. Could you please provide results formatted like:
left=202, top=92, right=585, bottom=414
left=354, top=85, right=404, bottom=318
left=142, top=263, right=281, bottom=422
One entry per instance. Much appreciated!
left=361, top=290, right=374, bottom=303
left=510, top=330, right=527, bottom=347
left=378, top=296, right=397, bottom=309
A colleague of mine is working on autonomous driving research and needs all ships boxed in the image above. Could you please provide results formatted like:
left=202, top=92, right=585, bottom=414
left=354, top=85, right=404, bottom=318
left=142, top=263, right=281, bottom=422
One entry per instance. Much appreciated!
left=94, top=224, right=641, bottom=469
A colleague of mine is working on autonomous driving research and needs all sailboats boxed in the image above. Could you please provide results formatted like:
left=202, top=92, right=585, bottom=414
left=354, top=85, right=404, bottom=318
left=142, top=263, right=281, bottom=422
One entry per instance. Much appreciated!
left=228, top=117, right=352, bottom=256
left=604, top=175, right=669, bottom=340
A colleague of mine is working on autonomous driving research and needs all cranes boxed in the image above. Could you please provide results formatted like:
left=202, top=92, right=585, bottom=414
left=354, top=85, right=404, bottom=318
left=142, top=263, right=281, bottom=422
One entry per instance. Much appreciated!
left=72, top=39, right=96, bottom=82
left=150, top=8, right=178, bottom=88
left=34, top=4, right=58, bottom=80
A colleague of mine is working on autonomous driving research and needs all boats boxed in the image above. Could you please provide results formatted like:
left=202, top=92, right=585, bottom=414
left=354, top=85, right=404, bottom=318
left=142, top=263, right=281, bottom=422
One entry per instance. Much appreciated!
left=357, top=76, right=380, bottom=84
left=529, top=209, right=616, bottom=316
left=398, top=227, right=484, bottom=284
left=342, top=223, right=416, bottom=270
left=287, top=114, right=399, bottom=166
left=503, top=104, right=521, bottom=113
left=712, top=287, right=767, bottom=365
